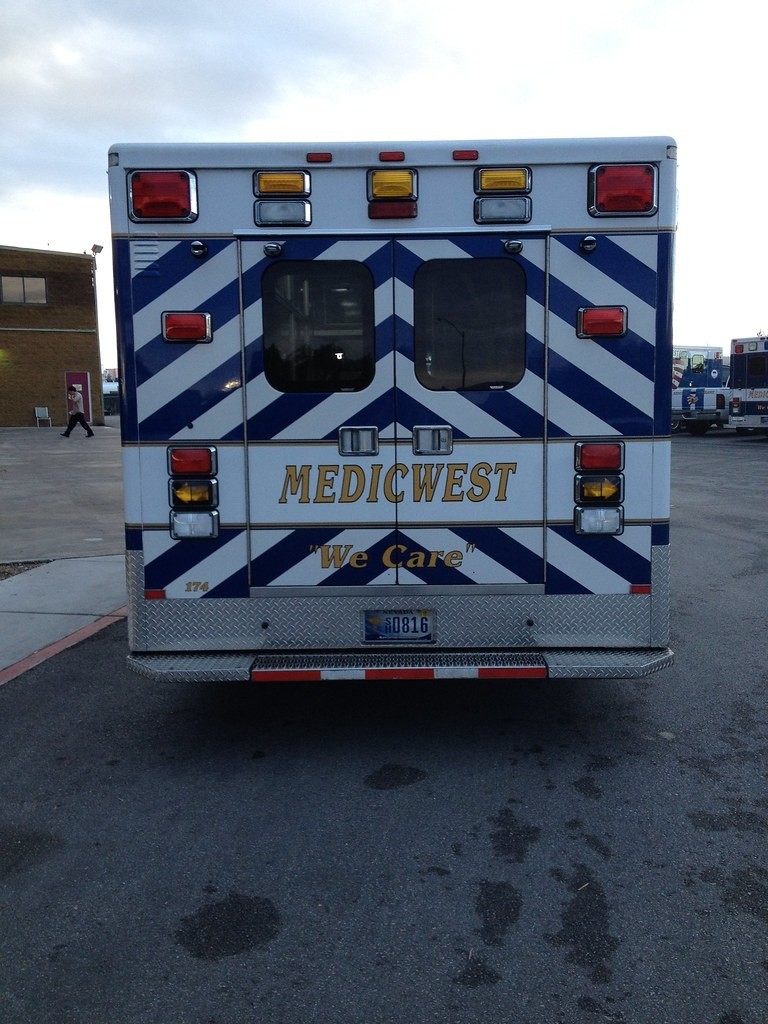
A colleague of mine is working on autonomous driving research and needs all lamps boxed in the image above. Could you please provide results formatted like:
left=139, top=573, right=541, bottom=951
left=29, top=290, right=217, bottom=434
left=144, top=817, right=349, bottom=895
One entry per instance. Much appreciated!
left=91, top=244, right=103, bottom=258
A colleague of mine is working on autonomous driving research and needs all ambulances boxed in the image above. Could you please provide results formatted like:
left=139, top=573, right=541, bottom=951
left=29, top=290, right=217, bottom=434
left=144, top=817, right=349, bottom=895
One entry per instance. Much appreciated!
left=104, top=135, right=677, bottom=686
left=729, top=335, right=768, bottom=433
left=671, top=345, right=729, bottom=432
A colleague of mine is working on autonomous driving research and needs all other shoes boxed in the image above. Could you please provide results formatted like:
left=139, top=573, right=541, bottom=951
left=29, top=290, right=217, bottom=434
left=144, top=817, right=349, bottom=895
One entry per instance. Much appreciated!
left=60, top=433, right=69, bottom=437
left=85, top=434, right=94, bottom=437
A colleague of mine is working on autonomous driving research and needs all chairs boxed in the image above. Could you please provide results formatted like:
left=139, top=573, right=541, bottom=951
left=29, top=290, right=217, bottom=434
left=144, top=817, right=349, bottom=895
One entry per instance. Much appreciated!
left=35, top=407, right=52, bottom=427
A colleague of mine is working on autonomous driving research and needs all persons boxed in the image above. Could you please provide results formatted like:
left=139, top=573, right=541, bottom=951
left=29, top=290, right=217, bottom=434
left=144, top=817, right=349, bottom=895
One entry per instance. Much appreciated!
left=59, top=385, right=95, bottom=438
